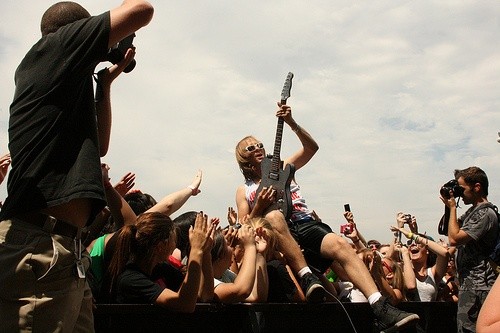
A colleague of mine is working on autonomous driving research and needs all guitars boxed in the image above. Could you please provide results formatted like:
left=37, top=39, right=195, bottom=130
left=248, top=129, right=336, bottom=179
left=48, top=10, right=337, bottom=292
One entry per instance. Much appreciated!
left=254, top=72, right=296, bottom=221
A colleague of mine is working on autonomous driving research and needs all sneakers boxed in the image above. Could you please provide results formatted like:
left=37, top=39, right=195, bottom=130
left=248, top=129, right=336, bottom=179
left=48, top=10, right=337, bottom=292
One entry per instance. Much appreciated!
left=300, top=272, right=326, bottom=303
left=371, top=297, right=420, bottom=333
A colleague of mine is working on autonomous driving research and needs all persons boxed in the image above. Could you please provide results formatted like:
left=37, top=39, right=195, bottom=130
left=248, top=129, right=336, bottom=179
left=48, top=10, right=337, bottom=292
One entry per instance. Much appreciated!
left=235, top=101, right=419, bottom=333
left=476, top=274, right=500, bottom=333
left=1, top=157, right=463, bottom=304
left=0, top=2, right=154, bottom=333
left=438, top=167, right=500, bottom=333
left=92, top=211, right=221, bottom=315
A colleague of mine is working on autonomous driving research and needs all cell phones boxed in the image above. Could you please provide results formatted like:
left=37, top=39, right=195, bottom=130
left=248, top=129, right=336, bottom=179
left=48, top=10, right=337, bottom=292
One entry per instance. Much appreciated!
left=344, top=204, right=350, bottom=212
left=230, top=236, right=239, bottom=249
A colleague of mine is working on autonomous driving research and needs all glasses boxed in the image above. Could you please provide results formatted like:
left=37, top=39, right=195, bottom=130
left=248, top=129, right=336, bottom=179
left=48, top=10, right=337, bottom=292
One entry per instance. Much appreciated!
left=245, top=143, right=264, bottom=152
left=368, top=244, right=380, bottom=248
left=406, top=239, right=421, bottom=245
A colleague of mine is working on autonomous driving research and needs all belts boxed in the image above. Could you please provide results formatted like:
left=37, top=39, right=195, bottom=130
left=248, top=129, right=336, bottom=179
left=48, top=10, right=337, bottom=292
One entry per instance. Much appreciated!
left=14, top=210, right=88, bottom=243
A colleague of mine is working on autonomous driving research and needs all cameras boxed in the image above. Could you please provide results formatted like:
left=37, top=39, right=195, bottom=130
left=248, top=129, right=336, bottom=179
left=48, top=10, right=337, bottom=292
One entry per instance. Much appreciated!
left=402, top=215, right=412, bottom=224
left=100, top=32, right=136, bottom=73
left=339, top=223, right=353, bottom=234
left=440, top=179, right=460, bottom=198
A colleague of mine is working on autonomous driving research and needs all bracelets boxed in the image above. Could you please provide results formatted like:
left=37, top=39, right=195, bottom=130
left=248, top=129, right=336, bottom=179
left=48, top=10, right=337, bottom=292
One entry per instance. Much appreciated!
left=292, top=124, right=301, bottom=133
